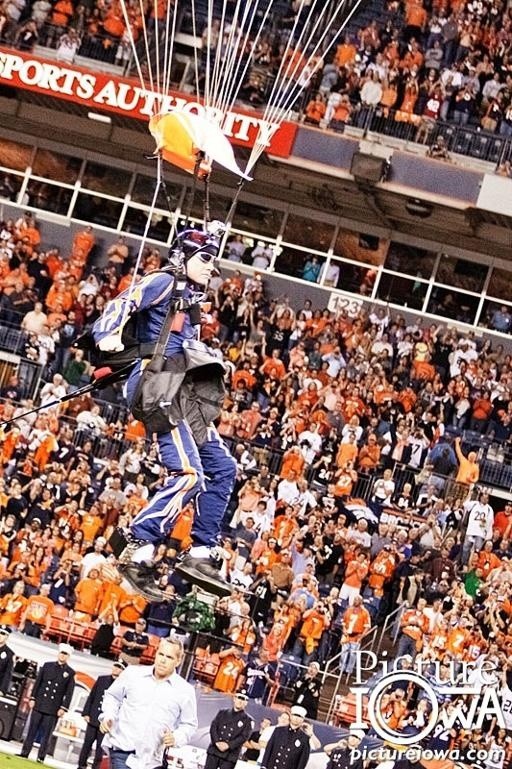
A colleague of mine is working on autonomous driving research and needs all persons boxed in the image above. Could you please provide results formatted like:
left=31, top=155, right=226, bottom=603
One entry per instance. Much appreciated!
left=2, top=214, right=512, bottom=769
left=1, top=0, right=512, bottom=175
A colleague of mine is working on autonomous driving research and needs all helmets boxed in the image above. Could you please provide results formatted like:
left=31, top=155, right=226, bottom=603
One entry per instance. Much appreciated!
left=168, top=229, right=219, bottom=264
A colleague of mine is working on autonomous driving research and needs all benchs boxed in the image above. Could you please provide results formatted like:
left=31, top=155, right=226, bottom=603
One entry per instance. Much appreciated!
left=439, top=424, right=512, bottom=458
left=407, top=119, right=512, bottom=165
left=51, top=711, right=261, bottom=769
left=43, top=532, right=384, bottom=707
left=332, top=691, right=511, bottom=765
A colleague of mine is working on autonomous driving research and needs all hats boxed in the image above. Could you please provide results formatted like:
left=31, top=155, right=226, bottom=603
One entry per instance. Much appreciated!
left=290, top=705, right=307, bottom=718
left=0, top=625, right=12, bottom=635
left=114, top=658, right=128, bottom=670
left=350, top=729, right=365, bottom=740
left=409, top=742, right=422, bottom=749
left=236, top=689, right=249, bottom=701
left=59, top=643, right=74, bottom=655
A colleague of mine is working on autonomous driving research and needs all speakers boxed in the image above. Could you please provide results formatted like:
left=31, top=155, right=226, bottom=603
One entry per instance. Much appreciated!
left=350, top=152, right=386, bottom=182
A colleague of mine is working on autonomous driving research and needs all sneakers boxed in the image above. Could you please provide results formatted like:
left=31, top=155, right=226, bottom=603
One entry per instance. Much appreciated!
left=175, top=545, right=231, bottom=596
left=118, top=541, right=162, bottom=602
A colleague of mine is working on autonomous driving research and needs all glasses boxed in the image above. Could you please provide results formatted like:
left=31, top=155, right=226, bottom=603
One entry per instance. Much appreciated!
left=198, top=253, right=217, bottom=262
